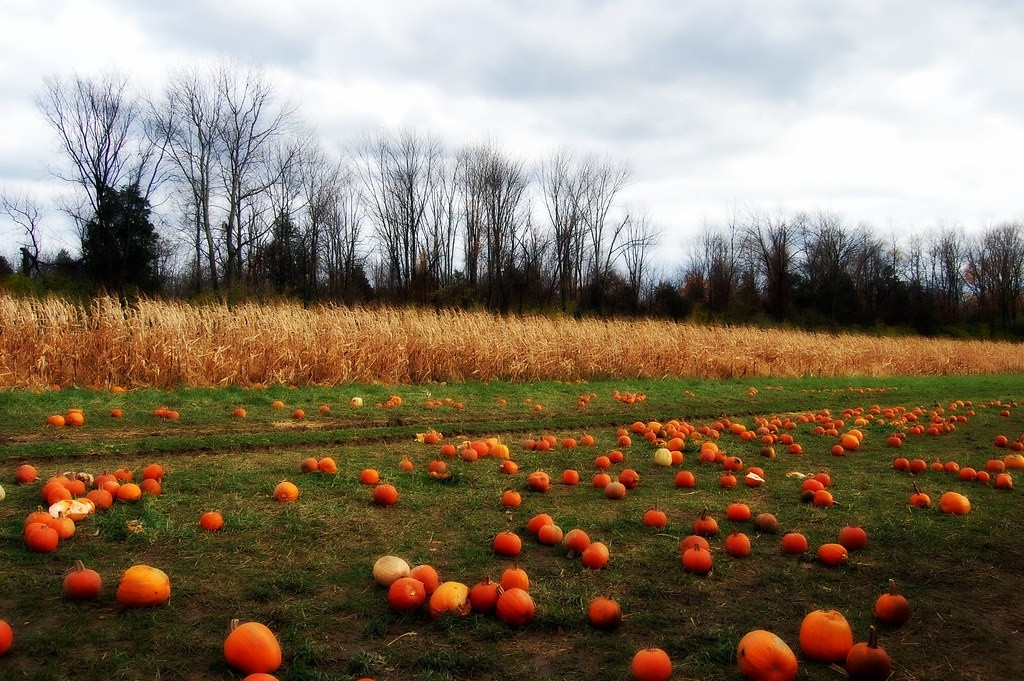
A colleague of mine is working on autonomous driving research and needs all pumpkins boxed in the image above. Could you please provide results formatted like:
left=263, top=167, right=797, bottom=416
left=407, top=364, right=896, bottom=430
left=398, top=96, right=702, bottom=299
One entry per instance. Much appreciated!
left=0, top=385, right=1024, bottom=681
